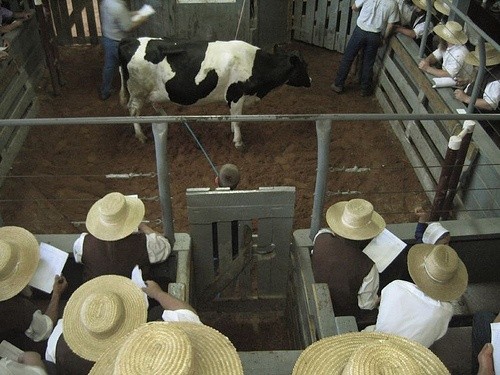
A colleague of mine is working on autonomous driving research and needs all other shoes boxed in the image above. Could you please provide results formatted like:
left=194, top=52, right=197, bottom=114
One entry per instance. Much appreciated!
left=101, top=93, right=109, bottom=101
left=361, top=90, right=366, bottom=96
left=331, top=83, right=343, bottom=93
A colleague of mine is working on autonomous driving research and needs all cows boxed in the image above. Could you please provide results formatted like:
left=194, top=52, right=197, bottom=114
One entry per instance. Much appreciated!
left=117, top=37, right=312, bottom=151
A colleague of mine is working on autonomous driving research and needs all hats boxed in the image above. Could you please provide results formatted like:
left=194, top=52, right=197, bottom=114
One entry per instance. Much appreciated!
left=63, top=274, right=147, bottom=362
left=464, top=43, right=500, bottom=66
left=0, top=225, right=38, bottom=301
left=85, top=192, right=145, bottom=241
left=88, top=321, right=243, bottom=375
left=407, top=243, right=469, bottom=302
left=325, top=197, right=386, bottom=240
left=412, top=0, right=437, bottom=15
left=432, top=20, right=468, bottom=44
left=292, top=332, right=449, bottom=375
left=433, top=0, right=453, bottom=15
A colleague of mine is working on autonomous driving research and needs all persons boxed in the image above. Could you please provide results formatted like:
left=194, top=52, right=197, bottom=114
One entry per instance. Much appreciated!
left=415, top=207, right=451, bottom=244
left=89, top=321, right=245, bottom=375
left=361, top=243, right=468, bottom=348
left=418, top=21, right=500, bottom=128
left=0, top=225, right=68, bottom=360
left=99, top=0, right=148, bottom=101
left=0, top=0, right=48, bottom=60
left=292, top=332, right=451, bottom=375
left=0, top=352, right=48, bottom=375
left=391, top=0, right=453, bottom=57
left=212, top=163, right=252, bottom=278
left=478, top=313, right=500, bottom=375
left=73, top=192, right=171, bottom=310
left=330, top=0, right=400, bottom=96
left=45, top=275, right=200, bottom=375
left=311, top=199, right=386, bottom=320
